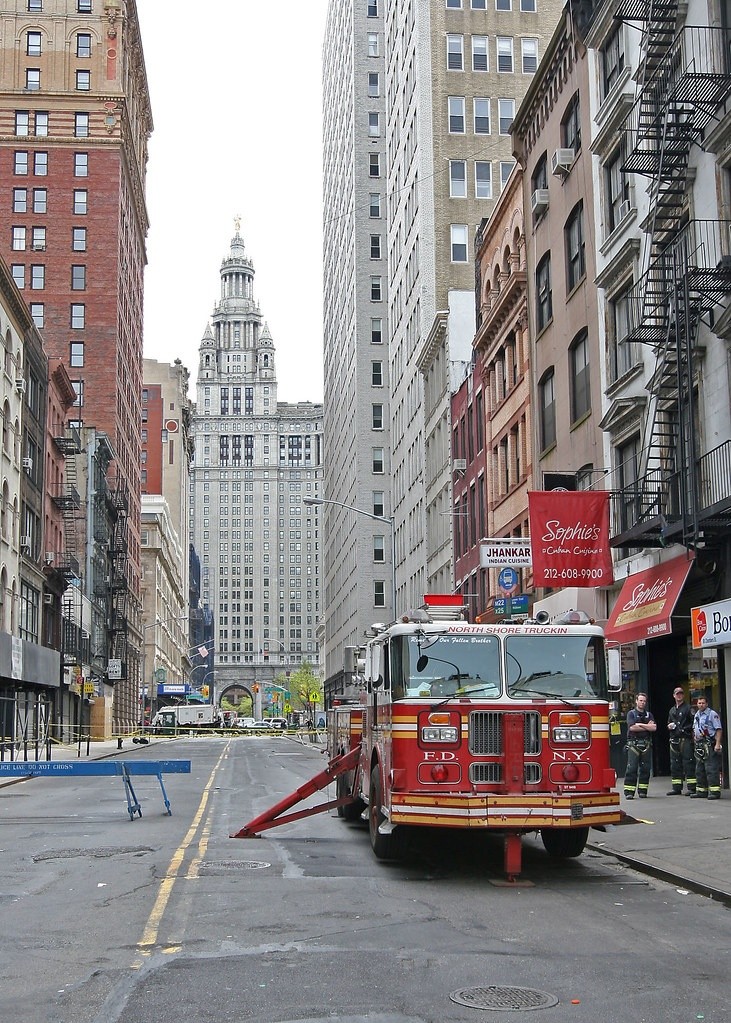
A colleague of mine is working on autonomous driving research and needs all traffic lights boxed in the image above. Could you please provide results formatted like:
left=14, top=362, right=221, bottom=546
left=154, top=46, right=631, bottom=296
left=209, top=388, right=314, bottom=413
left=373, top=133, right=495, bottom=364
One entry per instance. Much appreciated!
left=201, top=686, right=210, bottom=698
left=252, top=684, right=259, bottom=694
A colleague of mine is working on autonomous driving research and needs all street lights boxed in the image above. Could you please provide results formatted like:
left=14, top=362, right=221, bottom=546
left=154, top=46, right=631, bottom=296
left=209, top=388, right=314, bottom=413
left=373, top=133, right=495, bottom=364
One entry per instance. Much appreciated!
left=141, top=614, right=190, bottom=734
left=302, top=495, right=396, bottom=623
left=263, top=637, right=289, bottom=720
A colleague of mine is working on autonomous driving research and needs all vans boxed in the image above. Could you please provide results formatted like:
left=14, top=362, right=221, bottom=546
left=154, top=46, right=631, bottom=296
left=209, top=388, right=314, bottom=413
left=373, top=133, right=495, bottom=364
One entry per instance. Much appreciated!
left=223, top=710, right=238, bottom=724
left=233, top=716, right=300, bottom=730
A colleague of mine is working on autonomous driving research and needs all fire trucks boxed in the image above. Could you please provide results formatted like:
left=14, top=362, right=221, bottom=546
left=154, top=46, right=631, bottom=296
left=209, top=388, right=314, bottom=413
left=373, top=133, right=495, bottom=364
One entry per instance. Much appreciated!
left=229, top=594, right=655, bottom=889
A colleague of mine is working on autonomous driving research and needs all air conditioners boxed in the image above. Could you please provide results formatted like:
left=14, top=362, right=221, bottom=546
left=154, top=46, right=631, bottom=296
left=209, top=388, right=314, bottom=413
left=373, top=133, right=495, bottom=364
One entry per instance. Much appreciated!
left=21, top=536, right=30, bottom=548
left=551, top=148, right=575, bottom=175
left=45, top=552, right=54, bottom=562
left=45, top=594, right=53, bottom=604
left=23, top=458, right=32, bottom=470
left=15, top=378, right=26, bottom=393
left=531, top=189, right=549, bottom=214
left=83, top=632, right=89, bottom=639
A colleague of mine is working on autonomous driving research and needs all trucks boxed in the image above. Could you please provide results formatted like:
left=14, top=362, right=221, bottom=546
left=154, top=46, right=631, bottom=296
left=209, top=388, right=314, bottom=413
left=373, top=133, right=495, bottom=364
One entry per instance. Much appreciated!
left=149, top=705, right=218, bottom=733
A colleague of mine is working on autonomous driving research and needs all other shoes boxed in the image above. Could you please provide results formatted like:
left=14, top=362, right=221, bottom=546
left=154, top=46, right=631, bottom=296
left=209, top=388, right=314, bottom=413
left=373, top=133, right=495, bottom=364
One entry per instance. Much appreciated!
left=708, top=794, right=720, bottom=799
left=685, top=790, right=696, bottom=796
left=639, top=792, right=646, bottom=798
left=666, top=789, right=681, bottom=795
left=690, top=792, right=708, bottom=798
left=626, top=794, right=633, bottom=799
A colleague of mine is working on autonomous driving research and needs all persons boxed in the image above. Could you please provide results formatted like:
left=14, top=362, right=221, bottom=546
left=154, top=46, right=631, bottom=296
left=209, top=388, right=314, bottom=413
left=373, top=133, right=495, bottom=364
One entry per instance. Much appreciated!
left=624, top=693, right=657, bottom=799
left=666, top=688, right=697, bottom=796
left=690, top=697, right=723, bottom=800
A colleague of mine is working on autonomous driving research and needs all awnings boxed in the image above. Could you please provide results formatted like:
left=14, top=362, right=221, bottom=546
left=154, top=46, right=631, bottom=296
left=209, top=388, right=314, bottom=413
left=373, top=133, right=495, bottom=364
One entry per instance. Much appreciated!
left=604, top=551, right=696, bottom=649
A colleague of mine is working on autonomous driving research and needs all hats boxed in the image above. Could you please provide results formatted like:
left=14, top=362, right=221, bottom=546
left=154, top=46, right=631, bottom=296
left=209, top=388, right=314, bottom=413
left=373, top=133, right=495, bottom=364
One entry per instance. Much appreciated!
left=674, top=687, right=684, bottom=695
